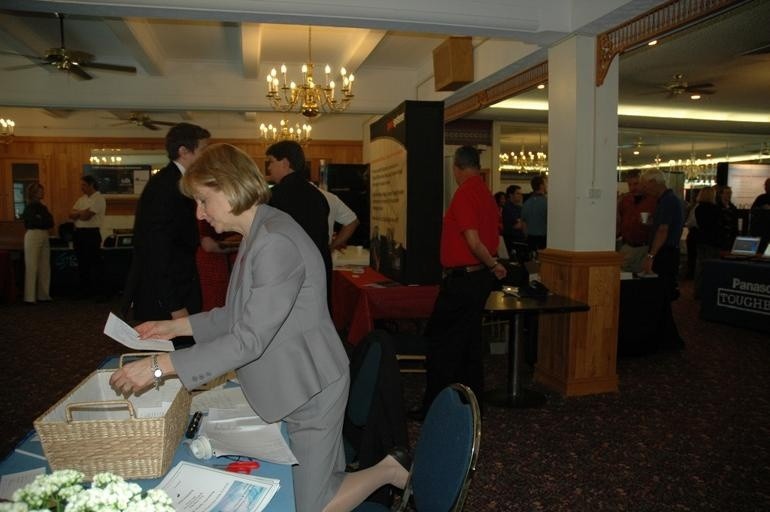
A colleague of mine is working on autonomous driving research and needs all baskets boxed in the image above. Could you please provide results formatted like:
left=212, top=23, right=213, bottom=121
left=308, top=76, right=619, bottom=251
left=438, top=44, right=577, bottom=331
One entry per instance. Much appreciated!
left=32, top=350, right=193, bottom=484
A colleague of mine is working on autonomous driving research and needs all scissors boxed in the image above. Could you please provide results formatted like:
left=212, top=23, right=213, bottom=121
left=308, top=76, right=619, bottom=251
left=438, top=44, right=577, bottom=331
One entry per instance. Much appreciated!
left=212, top=461, right=260, bottom=475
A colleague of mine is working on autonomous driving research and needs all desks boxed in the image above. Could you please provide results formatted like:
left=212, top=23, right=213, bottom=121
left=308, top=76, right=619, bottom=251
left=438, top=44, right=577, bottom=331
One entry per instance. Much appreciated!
left=616, top=277, right=685, bottom=359
left=0, top=357, right=299, bottom=512
left=330, top=266, right=442, bottom=344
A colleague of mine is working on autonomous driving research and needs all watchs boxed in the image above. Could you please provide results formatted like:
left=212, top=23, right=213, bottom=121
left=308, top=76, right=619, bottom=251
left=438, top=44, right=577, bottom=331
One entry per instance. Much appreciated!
left=150, top=353, right=165, bottom=392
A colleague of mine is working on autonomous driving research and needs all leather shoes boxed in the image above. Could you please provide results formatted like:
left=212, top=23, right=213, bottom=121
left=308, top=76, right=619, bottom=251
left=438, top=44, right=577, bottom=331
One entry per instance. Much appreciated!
left=385, top=438, right=420, bottom=473
left=406, top=405, right=428, bottom=421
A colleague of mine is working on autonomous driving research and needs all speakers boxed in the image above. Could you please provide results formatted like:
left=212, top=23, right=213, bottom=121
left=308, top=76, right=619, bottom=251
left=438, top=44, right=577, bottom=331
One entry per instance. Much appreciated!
left=431, top=35, right=474, bottom=93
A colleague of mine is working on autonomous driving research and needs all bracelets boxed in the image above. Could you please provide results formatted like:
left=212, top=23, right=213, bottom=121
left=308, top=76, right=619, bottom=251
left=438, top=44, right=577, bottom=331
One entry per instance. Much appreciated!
left=488, top=262, right=498, bottom=269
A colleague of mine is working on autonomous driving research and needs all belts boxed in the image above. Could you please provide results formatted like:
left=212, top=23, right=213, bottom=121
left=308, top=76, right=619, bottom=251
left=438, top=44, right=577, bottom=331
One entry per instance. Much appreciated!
left=442, top=263, right=489, bottom=278
left=27, top=227, right=48, bottom=230
left=74, top=226, right=100, bottom=231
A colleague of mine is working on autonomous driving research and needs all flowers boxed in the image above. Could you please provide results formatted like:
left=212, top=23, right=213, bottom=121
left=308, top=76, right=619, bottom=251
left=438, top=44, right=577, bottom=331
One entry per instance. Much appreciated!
left=0, top=468, right=175, bottom=511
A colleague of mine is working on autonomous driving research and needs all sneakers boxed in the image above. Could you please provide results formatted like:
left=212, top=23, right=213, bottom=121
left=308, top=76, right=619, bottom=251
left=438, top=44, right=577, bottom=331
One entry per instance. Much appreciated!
left=662, top=336, right=684, bottom=351
left=24, top=298, right=54, bottom=306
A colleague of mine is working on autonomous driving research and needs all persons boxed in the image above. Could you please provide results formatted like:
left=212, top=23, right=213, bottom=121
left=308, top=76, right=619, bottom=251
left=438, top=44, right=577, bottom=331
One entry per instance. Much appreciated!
left=68, top=175, right=106, bottom=302
left=616, top=167, right=769, bottom=354
left=22, top=180, right=55, bottom=303
left=266, top=139, right=332, bottom=322
left=129, top=123, right=220, bottom=344
left=109, top=143, right=413, bottom=512
left=306, top=176, right=360, bottom=255
left=494, top=175, right=548, bottom=270
left=427, top=146, right=508, bottom=403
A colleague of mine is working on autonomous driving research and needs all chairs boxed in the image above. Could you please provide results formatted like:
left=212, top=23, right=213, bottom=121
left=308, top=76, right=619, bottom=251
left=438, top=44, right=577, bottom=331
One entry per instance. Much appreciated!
left=352, top=382, right=483, bottom=511
left=346, top=341, right=384, bottom=470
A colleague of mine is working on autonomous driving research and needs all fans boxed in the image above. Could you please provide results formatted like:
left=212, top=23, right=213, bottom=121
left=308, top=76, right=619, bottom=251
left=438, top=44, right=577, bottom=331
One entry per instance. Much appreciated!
left=99, top=112, right=177, bottom=131
left=639, top=72, right=718, bottom=99
left=0, top=12, right=136, bottom=80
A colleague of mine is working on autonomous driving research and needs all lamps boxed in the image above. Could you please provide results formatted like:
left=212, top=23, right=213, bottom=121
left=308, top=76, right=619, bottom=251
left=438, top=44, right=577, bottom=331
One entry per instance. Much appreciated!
left=0, top=118, right=15, bottom=144
left=499, top=140, right=546, bottom=174
left=258, top=25, right=355, bottom=146
left=656, top=141, right=718, bottom=183
